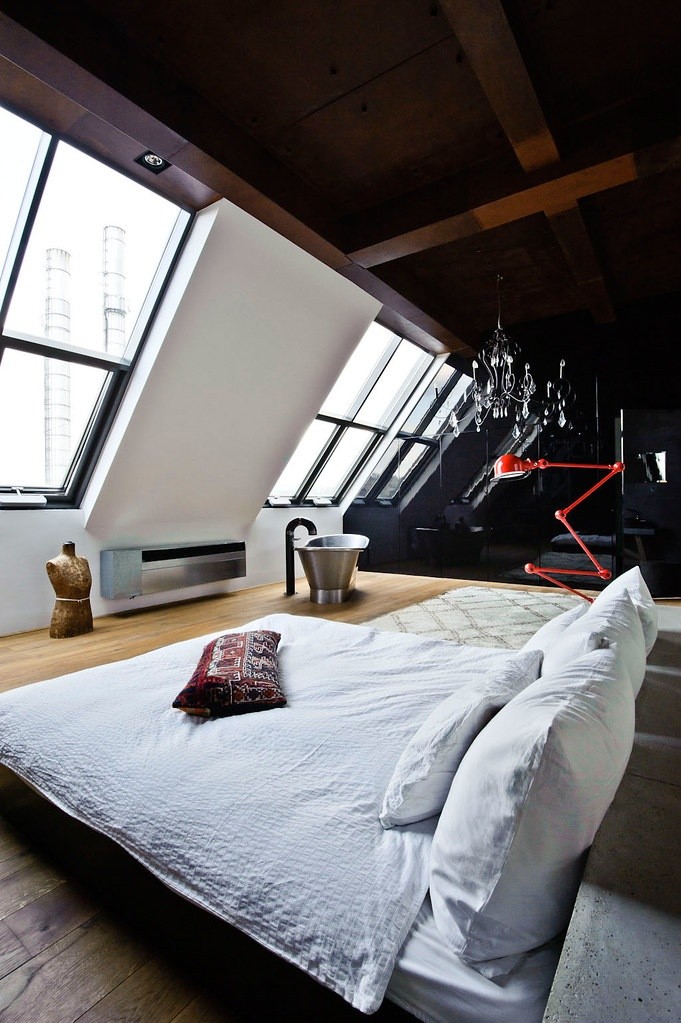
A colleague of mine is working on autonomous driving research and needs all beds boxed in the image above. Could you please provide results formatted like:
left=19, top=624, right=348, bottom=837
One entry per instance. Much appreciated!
left=550, top=530, right=616, bottom=553
left=0, top=614, right=584, bottom=1023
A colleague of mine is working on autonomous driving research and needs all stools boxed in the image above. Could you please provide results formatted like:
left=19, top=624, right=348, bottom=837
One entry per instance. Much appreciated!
left=625, top=528, right=657, bottom=561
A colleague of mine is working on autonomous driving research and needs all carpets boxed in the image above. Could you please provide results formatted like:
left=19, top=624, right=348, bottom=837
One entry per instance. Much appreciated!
left=499, top=548, right=614, bottom=587
left=354, top=586, right=586, bottom=650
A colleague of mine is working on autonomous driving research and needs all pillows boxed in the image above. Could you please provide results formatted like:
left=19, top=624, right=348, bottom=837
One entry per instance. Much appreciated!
left=380, top=649, right=544, bottom=829
left=429, top=638, right=634, bottom=984
left=588, top=566, right=658, bottom=657
left=525, top=601, right=589, bottom=651
left=542, top=588, right=647, bottom=703
left=172, top=630, right=287, bottom=718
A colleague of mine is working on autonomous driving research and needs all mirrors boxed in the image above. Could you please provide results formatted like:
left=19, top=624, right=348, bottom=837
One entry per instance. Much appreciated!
left=629, top=451, right=667, bottom=483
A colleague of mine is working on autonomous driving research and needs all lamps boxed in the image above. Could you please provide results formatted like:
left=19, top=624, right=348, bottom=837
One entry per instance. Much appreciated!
left=450, top=274, right=576, bottom=441
left=490, top=454, right=625, bottom=602
